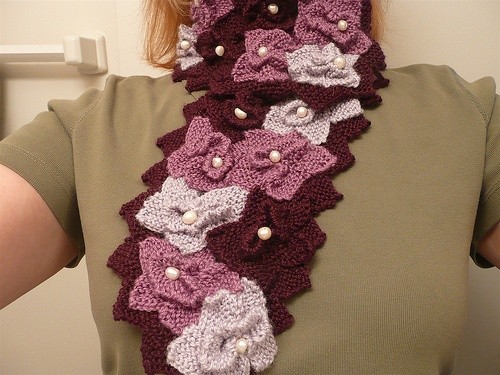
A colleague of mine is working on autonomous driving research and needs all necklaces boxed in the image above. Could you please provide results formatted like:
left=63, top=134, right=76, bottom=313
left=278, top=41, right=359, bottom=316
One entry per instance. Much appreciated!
left=179, top=77, right=200, bottom=103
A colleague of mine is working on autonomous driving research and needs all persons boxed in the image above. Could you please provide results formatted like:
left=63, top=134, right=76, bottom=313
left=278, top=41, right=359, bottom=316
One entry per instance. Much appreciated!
left=0, top=0, right=500, bottom=375
